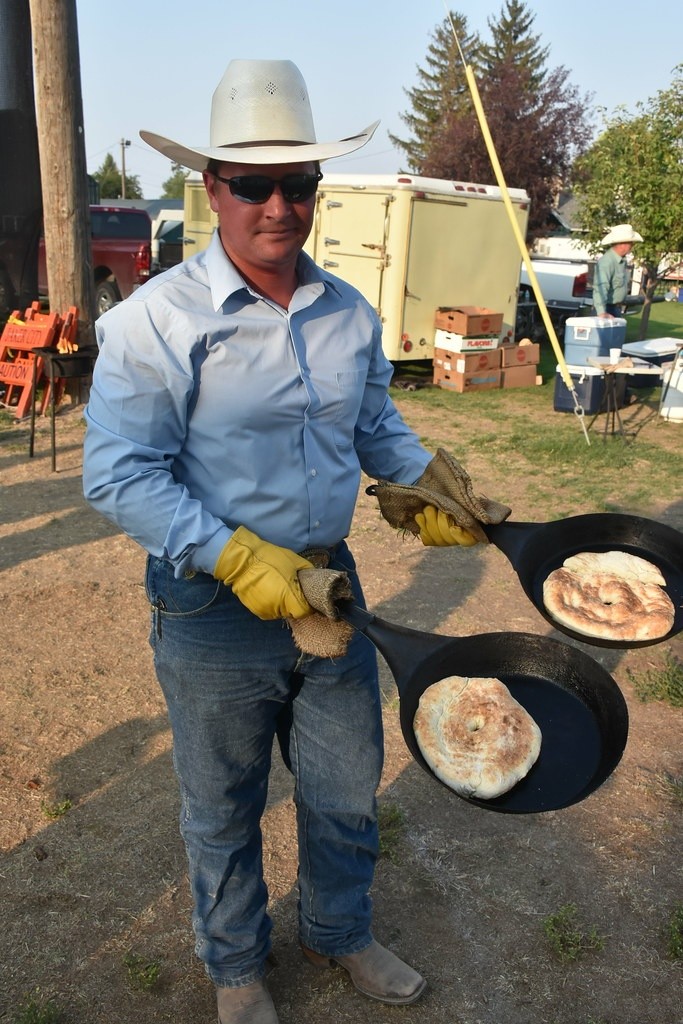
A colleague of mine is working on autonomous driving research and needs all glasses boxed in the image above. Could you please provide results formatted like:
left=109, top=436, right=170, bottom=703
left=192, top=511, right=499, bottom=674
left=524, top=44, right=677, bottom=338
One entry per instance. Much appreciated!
left=207, top=164, right=323, bottom=204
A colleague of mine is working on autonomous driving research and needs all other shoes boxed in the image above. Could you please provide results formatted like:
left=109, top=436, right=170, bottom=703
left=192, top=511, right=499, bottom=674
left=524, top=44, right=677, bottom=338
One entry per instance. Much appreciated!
left=624, top=393, right=638, bottom=404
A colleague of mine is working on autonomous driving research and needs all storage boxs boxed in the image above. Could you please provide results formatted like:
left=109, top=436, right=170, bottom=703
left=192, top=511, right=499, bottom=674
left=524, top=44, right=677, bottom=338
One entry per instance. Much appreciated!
left=432, top=306, right=543, bottom=393
left=553, top=316, right=683, bottom=415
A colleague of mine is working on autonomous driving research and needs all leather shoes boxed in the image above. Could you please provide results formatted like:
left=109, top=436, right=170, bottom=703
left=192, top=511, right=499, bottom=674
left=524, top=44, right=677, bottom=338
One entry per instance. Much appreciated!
left=300, top=939, right=428, bottom=1005
left=214, top=976, right=279, bottom=1024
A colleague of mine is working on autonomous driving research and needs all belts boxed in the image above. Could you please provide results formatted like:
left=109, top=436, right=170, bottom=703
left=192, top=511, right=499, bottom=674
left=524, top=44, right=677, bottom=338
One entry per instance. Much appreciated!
left=298, top=543, right=337, bottom=570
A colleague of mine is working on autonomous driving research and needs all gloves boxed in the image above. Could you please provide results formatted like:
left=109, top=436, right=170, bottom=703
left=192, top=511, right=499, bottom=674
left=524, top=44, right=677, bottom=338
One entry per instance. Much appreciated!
left=415, top=505, right=479, bottom=547
left=599, top=356, right=633, bottom=374
left=213, top=525, right=317, bottom=621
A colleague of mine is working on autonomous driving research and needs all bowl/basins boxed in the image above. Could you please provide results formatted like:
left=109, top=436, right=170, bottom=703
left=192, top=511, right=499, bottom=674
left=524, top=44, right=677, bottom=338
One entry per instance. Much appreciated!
left=477, top=511, right=683, bottom=650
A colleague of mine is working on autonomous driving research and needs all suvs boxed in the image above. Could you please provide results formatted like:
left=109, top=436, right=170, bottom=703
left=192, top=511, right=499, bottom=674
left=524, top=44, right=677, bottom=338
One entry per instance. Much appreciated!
left=37, top=204, right=152, bottom=321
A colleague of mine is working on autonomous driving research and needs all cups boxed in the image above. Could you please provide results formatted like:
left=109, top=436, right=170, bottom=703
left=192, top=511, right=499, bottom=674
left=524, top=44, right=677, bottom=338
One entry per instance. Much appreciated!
left=609, top=347, right=621, bottom=365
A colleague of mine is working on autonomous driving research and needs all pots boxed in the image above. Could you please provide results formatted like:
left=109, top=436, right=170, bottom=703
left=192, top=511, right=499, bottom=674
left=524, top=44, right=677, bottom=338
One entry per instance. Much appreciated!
left=332, top=593, right=629, bottom=814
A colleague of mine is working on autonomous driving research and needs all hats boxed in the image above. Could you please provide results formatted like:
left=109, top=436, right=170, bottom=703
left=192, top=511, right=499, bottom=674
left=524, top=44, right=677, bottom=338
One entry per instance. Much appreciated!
left=140, top=58, right=382, bottom=173
left=601, top=224, right=644, bottom=246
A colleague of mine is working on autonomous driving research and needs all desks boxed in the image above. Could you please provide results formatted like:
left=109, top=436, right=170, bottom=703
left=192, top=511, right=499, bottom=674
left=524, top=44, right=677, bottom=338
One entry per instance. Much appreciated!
left=585, top=357, right=663, bottom=447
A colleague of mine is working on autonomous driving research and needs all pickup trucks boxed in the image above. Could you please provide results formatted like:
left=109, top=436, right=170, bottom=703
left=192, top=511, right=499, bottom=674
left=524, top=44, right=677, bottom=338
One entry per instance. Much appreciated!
left=514, top=252, right=645, bottom=344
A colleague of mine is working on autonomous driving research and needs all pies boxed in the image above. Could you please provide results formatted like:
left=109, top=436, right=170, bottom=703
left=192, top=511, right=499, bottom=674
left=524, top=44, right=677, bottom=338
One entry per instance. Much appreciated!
left=543, top=551, right=675, bottom=641
left=413, top=675, right=541, bottom=800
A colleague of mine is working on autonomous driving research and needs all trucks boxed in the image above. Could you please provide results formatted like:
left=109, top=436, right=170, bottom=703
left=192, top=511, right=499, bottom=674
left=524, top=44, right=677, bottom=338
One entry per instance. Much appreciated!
left=148, top=171, right=530, bottom=369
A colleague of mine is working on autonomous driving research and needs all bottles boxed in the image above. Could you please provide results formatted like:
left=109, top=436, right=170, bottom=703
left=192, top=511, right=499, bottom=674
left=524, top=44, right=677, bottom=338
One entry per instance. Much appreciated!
left=525, top=290, right=529, bottom=303
left=675, top=348, right=683, bottom=371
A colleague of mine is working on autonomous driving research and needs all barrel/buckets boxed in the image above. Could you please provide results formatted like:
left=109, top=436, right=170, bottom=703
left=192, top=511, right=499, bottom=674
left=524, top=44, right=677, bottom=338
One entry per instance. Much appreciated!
left=678, top=288, right=683, bottom=303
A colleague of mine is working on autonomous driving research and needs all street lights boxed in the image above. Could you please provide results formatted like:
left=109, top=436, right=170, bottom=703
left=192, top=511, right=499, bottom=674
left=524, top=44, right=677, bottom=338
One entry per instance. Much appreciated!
left=121, top=137, right=131, bottom=199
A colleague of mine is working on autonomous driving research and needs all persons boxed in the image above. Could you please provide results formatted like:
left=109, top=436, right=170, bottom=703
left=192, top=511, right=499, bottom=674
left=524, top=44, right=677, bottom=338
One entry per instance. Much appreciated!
left=593, top=224, right=643, bottom=318
left=642, top=268, right=651, bottom=294
left=84, top=57, right=490, bottom=1023
